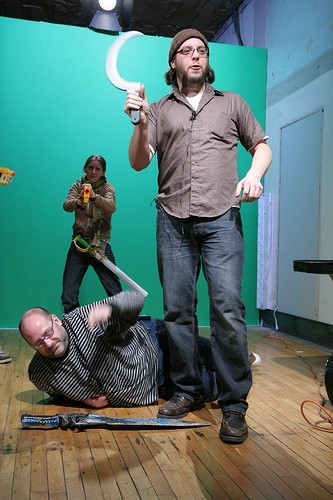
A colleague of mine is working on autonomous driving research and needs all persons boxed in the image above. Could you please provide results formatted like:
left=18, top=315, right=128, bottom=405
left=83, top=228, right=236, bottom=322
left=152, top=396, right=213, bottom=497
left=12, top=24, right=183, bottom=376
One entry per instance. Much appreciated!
left=60, top=155, right=122, bottom=315
left=124, top=29, right=272, bottom=444
left=18, top=290, right=261, bottom=407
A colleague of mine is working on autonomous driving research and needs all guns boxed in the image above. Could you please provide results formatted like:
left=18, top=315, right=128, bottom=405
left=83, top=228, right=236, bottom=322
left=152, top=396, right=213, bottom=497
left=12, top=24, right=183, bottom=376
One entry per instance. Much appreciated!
left=0, top=168, right=17, bottom=186
left=80, top=185, right=91, bottom=206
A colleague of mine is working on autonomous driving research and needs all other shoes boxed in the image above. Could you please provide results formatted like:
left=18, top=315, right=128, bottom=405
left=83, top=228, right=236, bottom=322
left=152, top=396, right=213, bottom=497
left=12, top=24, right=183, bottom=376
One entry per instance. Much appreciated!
left=0, top=346, right=12, bottom=363
left=249, top=351, right=261, bottom=366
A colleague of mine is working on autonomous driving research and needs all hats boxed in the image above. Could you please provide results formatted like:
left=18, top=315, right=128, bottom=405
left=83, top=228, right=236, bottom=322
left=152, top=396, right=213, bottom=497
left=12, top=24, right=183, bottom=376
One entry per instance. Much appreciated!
left=169, top=29, right=209, bottom=62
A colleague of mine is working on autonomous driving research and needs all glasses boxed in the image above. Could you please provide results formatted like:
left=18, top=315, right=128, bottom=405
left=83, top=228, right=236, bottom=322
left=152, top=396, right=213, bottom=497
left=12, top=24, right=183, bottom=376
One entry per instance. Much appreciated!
left=30, top=318, right=54, bottom=350
left=176, top=46, right=208, bottom=57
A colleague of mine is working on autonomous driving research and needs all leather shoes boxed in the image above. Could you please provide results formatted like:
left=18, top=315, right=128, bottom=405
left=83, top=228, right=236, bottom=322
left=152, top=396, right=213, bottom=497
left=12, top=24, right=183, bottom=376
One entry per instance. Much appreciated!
left=219, top=411, right=248, bottom=443
left=157, top=395, right=205, bottom=419
